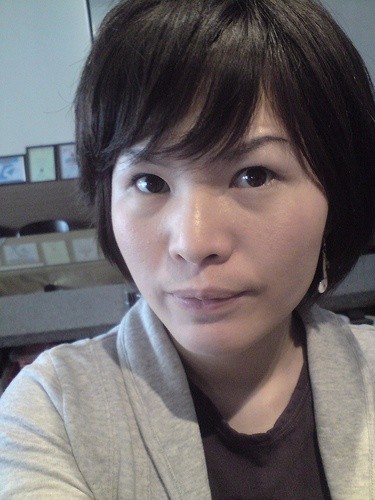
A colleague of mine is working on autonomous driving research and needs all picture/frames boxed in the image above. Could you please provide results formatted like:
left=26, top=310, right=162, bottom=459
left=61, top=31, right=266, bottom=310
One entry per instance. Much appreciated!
left=0, top=142, right=79, bottom=185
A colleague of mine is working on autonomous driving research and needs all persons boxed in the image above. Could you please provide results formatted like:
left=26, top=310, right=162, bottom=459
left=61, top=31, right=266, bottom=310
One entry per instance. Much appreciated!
left=0, top=0, right=375, bottom=500
